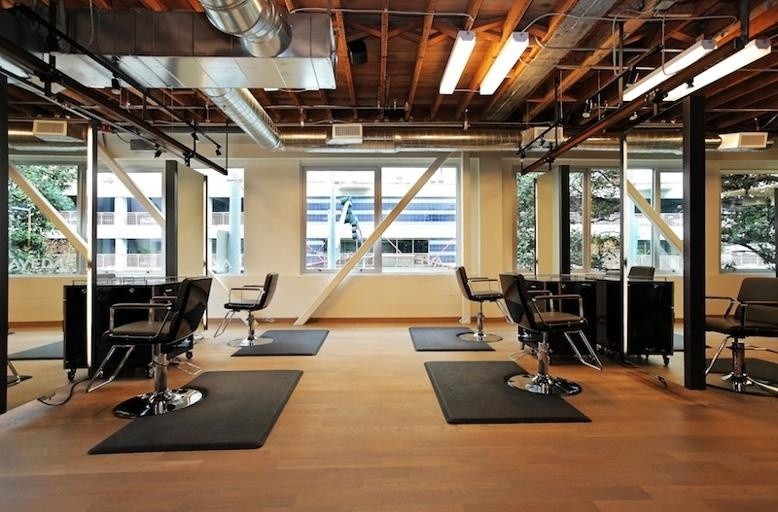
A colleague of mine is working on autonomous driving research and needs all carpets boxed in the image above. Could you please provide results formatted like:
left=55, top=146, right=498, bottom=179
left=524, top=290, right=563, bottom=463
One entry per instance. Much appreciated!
left=409, top=327, right=495, bottom=351
left=231, top=330, right=329, bottom=357
left=424, top=361, right=592, bottom=425
left=705, top=357, right=778, bottom=384
left=88, top=371, right=304, bottom=455
left=7, top=375, right=32, bottom=388
left=7, top=341, right=63, bottom=360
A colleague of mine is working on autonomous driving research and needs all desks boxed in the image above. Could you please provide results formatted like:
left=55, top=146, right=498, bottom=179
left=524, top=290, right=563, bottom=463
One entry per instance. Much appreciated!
left=596, top=276, right=675, bottom=365
left=517, top=276, right=599, bottom=355
left=63, top=285, right=96, bottom=381
left=93, top=280, right=182, bottom=379
left=102, top=278, right=197, bottom=364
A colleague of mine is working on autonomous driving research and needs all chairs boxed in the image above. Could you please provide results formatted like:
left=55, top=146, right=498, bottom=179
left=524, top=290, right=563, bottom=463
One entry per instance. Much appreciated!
left=85, top=278, right=213, bottom=420
left=214, top=273, right=281, bottom=347
left=499, top=274, right=605, bottom=397
left=456, top=266, right=514, bottom=342
left=705, top=277, right=778, bottom=396
left=628, top=266, right=656, bottom=280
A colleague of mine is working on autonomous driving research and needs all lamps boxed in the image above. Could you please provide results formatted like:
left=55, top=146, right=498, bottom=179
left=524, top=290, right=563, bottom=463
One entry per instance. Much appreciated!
left=438, top=30, right=529, bottom=96
left=623, top=39, right=772, bottom=103
